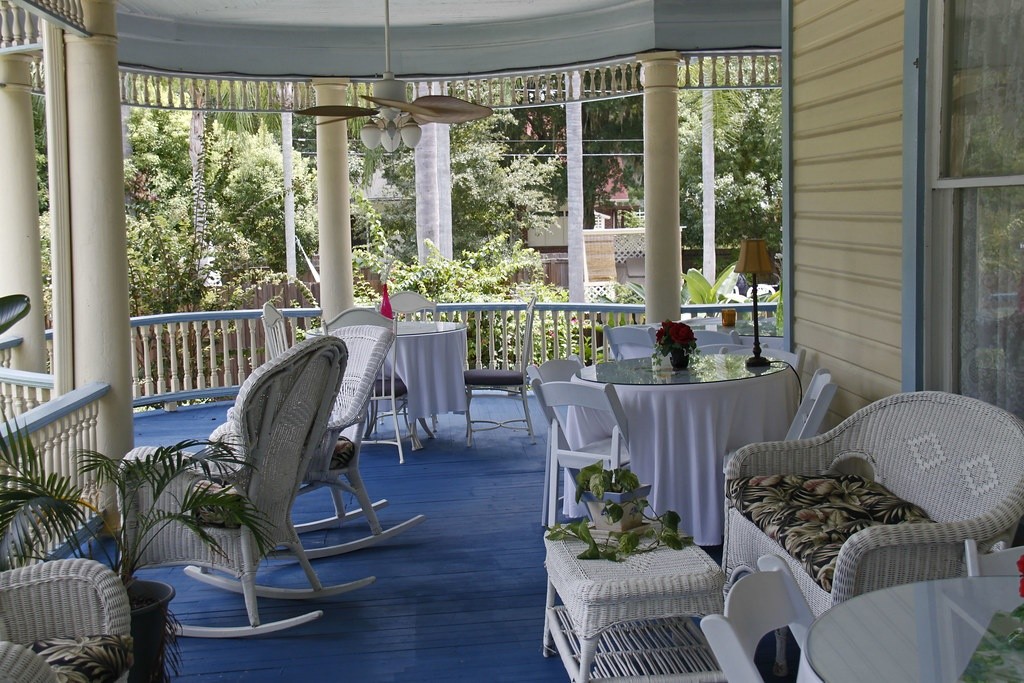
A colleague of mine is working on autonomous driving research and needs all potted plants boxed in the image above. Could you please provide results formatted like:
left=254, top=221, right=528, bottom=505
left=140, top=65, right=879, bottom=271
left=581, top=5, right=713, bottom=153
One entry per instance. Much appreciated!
left=545, top=458, right=697, bottom=560
left=0, top=406, right=277, bottom=683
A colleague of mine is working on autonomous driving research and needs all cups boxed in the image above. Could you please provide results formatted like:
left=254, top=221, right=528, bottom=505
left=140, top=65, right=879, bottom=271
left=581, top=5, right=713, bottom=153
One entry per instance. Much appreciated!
left=722, top=309, right=736, bottom=326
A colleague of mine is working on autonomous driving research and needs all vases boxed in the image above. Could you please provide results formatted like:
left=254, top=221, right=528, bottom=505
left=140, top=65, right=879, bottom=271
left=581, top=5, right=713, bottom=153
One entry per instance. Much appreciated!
left=380, top=284, right=392, bottom=318
left=671, top=349, right=691, bottom=368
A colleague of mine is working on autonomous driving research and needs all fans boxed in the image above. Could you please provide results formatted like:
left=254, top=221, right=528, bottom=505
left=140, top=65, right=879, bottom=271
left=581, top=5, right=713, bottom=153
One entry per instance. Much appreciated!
left=298, top=0, right=493, bottom=126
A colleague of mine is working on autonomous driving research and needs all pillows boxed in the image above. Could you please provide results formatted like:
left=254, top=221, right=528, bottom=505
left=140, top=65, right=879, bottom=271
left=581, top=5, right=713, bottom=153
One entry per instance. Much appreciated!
left=21, top=631, right=134, bottom=682
left=723, top=474, right=936, bottom=594
left=330, top=435, right=356, bottom=468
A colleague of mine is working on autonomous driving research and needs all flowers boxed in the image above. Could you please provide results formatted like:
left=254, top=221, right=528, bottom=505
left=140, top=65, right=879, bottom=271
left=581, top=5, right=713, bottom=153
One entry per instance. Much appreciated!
left=379, top=235, right=406, bottom=284
left=652, top=319, right=701, bottom=366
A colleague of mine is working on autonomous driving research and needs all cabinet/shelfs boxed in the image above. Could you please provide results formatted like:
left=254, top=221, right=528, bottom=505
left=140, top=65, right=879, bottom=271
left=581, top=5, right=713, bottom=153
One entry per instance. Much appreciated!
left=543, top=518, right=727, bottom=682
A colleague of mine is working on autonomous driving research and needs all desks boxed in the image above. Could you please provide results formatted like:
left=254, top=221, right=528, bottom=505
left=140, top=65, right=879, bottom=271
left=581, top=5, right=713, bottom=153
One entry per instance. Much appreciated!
left=801, top=577, right=1024, bottom=683
left=378, top=322, right=469, bottom=446
left=564, top=360, right=799, bottom=545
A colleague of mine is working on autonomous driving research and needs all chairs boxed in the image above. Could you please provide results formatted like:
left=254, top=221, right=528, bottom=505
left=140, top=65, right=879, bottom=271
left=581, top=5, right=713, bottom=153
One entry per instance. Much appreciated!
left=0, top=292, right=1023, bottom=683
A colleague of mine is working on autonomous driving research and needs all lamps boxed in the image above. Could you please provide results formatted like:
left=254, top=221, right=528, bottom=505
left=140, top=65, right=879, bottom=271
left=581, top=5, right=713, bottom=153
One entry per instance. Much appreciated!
left=359, top=107, right=422, bottom=152
left=735, top=238, right=774, bottom=366
